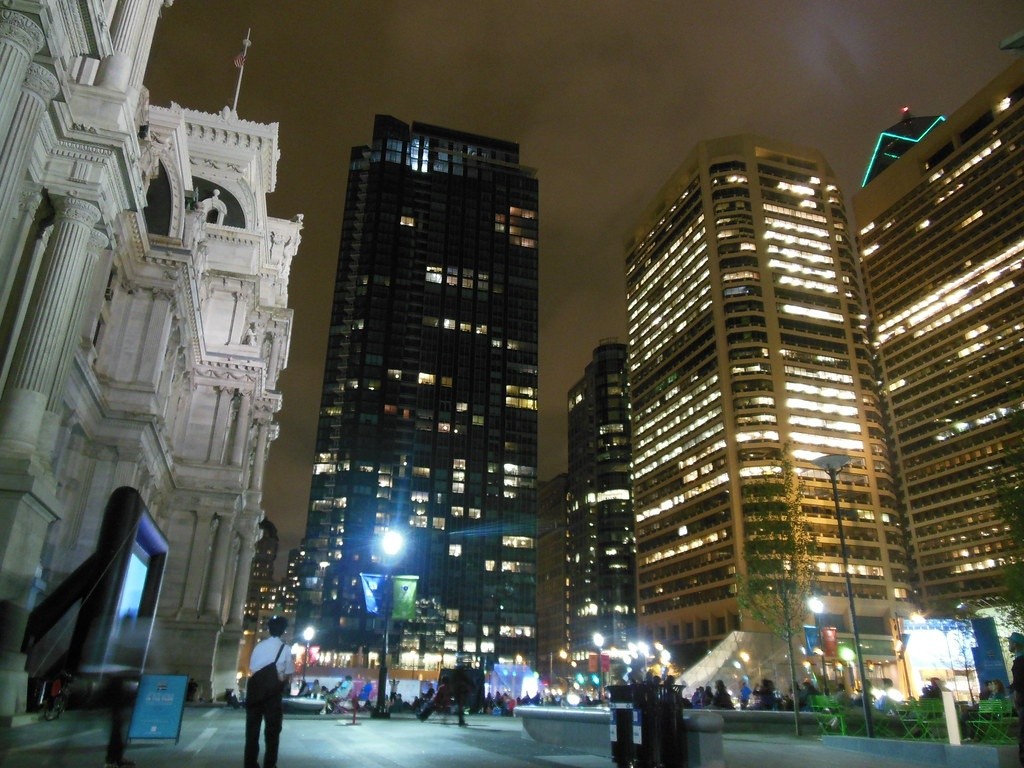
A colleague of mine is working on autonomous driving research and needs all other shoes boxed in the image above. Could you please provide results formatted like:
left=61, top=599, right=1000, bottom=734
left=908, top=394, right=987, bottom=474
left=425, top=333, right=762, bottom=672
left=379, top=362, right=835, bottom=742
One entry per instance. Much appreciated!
left=458, top=722, right=469, bottom=726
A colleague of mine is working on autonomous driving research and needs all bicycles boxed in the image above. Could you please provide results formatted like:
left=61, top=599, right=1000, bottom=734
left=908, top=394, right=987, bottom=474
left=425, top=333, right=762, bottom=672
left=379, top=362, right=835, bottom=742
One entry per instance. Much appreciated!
left=43, top=671, right=72, bottom=721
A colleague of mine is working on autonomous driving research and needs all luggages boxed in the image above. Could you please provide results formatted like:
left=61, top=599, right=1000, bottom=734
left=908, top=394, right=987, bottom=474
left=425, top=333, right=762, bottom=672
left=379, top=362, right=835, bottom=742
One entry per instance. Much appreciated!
left=417, top=701, right=436, bottom=723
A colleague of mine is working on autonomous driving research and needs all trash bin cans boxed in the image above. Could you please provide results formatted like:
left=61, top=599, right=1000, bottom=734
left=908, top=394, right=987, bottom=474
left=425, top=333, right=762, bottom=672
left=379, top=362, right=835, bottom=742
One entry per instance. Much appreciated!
left=605, top=681, right=686, bottom=768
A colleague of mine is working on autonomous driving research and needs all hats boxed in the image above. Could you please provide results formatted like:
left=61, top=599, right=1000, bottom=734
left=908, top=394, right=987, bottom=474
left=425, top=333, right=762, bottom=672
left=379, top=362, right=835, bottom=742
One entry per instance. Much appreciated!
left=1006, top=632, right=1024, bottom=645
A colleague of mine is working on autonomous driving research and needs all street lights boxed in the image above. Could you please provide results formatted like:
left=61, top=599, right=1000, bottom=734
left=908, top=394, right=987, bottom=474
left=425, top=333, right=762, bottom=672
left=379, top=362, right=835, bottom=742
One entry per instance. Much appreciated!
left=593, top=633, right=604, bottom=704
left=807, top=594, right=832, bottom=697
left=301, top=624, right=316, bottom=683
left=366, top=530, right=405, bottom=719
left=809, top=451, right=873, bottom=741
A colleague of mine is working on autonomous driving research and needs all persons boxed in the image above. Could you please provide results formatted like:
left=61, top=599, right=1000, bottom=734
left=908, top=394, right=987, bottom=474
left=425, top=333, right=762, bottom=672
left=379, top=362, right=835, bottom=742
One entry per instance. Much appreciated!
left=421, top=683, right=435, bottom=700
left=433, top=675, right=451, bottom=724
left=874, top=678, right=904, bottom=716
left=1006, top=631, right=1024, bottom=768
left=298, top=675, right=403, bottom=715
left=702, top=686, right=713, bottom=706
left=913, top=680, right=1005, bottom=742
left=188, top=677, right=199, bottom=702
left=738, top=682, right=752, bottom=710
left=749, top=679, right=818, bottom=712
left=822, top=678, right=873, bottom=733
left=712, top=680, right=736, bottom=710
left=241, top=617, right=296, bottom=768
left=484, top=690, right=540, bottom=717
left=641, top=673, right=704, bottom=711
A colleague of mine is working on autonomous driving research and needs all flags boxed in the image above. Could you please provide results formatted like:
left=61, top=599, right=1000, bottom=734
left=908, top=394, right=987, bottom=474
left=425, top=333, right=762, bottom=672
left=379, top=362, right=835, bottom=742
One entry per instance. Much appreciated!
left=233, top=48, right=248, bottom=68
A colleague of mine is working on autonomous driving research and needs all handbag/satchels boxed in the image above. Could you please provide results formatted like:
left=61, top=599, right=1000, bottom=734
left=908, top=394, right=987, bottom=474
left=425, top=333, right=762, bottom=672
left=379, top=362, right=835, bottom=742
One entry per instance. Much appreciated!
left=246, top=643, right=286, bottom=706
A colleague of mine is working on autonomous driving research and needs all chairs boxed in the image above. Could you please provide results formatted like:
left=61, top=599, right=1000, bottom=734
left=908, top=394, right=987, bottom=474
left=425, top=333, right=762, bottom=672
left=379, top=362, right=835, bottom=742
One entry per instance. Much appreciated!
left=431, top=698, right=452, bottom=724
left=807, top=695, right=853, bottom=736
left=852, top=697, right=1017, bottom=745
